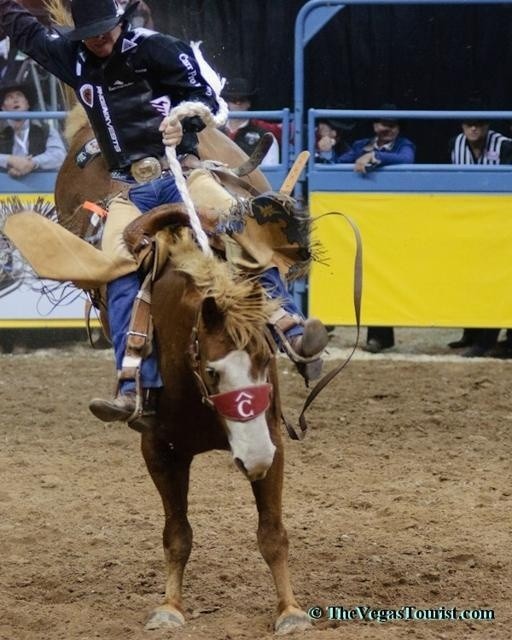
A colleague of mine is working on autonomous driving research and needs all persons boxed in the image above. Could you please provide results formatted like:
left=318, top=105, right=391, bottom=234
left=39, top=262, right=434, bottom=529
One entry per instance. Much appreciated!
left=212, top=77, right=284, bottom=167
left=310, top=118, right=362, bottom=339
left=439, top=99, right=512, bottom=357
left=334, top=103, right=415, bottom=355
left=487, top=327, right=511, bottom=360
left=1, top=0, right=330, bottom=436
left=0, top=77, right=72, bottom=356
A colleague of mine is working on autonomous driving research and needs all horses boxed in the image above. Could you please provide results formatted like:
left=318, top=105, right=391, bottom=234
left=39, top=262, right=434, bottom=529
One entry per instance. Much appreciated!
left=42, top=0, right=316, bottom=635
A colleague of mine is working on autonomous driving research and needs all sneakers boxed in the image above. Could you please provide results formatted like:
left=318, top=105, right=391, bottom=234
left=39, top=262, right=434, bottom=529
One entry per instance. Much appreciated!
left=448, top=335, right=497, bottom=356
left=363, top=337, right=394, bottom=352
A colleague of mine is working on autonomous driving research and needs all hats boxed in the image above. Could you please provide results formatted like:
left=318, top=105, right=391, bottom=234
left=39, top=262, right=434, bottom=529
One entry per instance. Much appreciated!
left=1, top=79, right=37, bottom=109
left=52, top=0, right=141, bottom=42
left=221, top=78, right=264, bottom=96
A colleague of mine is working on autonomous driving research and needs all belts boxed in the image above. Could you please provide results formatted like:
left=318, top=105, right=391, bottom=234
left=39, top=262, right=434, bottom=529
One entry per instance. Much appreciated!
left=113, top=150, right=187, bottom=184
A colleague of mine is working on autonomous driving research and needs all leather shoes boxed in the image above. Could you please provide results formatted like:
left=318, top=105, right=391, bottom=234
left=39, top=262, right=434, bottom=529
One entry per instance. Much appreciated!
left=286, top=319, right=328, bottom=380
left=89, top=397, right=156, bottom=432
